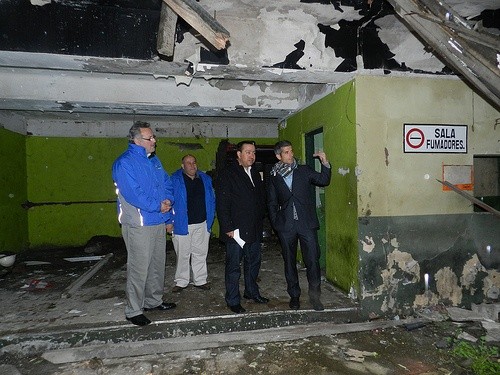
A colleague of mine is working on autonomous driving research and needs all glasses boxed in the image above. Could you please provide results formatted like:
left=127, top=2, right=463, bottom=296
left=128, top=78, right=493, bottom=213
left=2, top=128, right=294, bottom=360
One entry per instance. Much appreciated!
left=135, top=136, right=154, bottom=141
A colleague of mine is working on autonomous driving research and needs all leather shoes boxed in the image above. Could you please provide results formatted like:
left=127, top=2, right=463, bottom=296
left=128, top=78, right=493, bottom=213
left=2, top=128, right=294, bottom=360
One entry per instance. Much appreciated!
left=126, top=314, right=147, bottom=326
left=144, top=302, right=176, bottom=311
left=230, top=303, right=246, bottom=314
left=243, top=295, right=270, bottom=304
left=309, top=298, right=324, bottom=310
left=289, top=297, right=300, bottom=309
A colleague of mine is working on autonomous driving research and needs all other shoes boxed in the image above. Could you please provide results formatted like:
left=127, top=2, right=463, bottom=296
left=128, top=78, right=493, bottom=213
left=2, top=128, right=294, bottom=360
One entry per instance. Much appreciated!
left=193, top=284, right=210, bottom=291
left=171, top=286, right=183, bottom=292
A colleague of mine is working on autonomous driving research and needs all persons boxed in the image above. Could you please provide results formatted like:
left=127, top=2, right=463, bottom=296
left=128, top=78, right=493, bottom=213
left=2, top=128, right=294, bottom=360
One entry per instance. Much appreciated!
left=266, top=139, right=333, bottom=312
left=110, top=122, right=175, bottom=326
left=166, top=153, right=217, bottom=294
left=214, top=140, right=270, bottom=315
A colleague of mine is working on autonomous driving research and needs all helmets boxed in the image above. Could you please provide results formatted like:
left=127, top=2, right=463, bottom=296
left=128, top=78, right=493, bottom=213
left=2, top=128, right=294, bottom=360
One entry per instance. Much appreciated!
left=0, top=252, right=16, bottom=267
